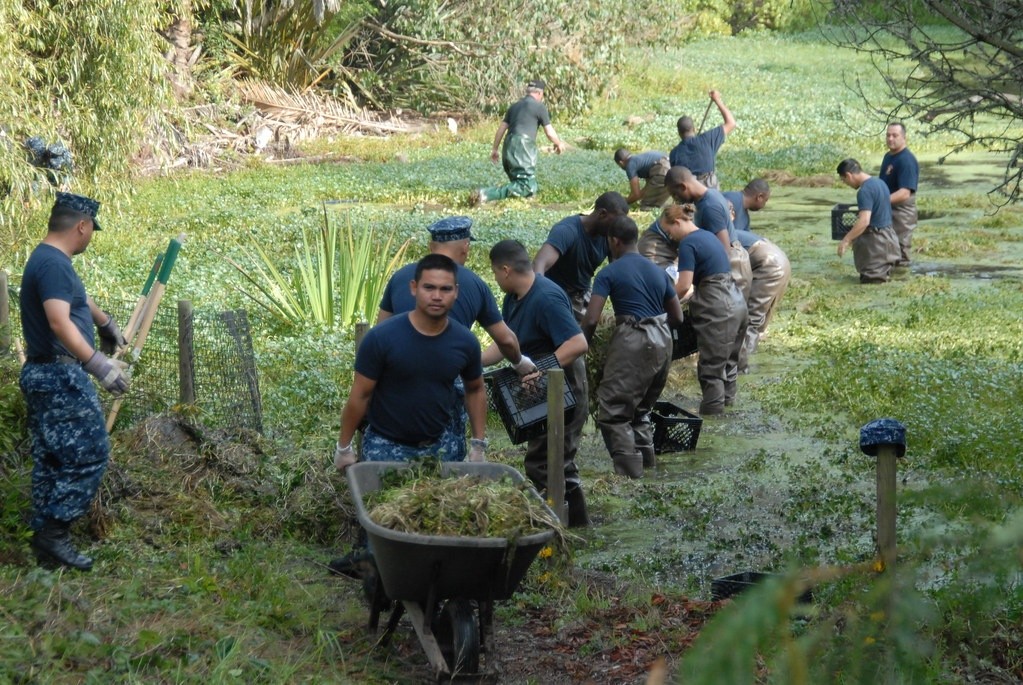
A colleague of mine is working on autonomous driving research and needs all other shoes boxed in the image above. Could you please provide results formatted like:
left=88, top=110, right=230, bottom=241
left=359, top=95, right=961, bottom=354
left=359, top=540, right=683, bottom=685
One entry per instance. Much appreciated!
left=470, top=186, right=486, bottom=209
left=327, top=557, right=363, bottom=579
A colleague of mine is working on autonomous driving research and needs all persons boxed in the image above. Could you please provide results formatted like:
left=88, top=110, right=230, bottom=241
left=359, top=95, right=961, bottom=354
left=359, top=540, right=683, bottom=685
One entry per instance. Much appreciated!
left=614, top=148, right=670, bottom=207
left=665, top=166, right=752, bottom=309
left=479, top=81, right=562, bottom=203
left=19, top=192, right=132, bottom=569
left=482, top=239, right=589, bottom=527
left=669, top=89, right=736, bottom=190
left=722, top=179, right=770, bottom=231
left=836, top=158, right=902, bottom=284
left=329, top=216, right=536, bottom=579
left=637, top=200, right=735, bottom=265
left=737, top=231, right=791, bottom=374
left=531, top=191, right=629, bottom=325
left=660, top=205, right=749, bottom=415
left=879, top=122, right=919, bottom=263
left=334, top=254, right=487, bottom=610
left=581, top=216, right=683, bottom=479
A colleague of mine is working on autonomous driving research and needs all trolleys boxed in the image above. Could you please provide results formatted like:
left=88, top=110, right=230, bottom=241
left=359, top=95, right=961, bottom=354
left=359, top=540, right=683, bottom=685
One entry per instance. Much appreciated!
left=344, top=458, right=559, bottom=685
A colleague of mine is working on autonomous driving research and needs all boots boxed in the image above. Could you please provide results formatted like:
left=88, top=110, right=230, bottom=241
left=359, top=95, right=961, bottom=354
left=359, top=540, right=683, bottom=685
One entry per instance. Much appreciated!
left=33, top=520, right=95, bottom=571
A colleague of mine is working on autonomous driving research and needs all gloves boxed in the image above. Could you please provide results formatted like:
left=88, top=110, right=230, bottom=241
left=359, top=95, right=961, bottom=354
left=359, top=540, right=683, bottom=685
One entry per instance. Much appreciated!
left=95, top=309, right=128, bottom=356
left=469, top=435, right=487, bottom=461
left=332, top=442, right=357, bottom=468
left=82, top=351, right=132, bottom=398
left=510, top=355, right=537, bottom=375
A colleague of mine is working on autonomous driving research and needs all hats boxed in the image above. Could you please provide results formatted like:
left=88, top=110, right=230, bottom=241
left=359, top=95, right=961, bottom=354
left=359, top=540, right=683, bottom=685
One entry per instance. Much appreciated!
left=429, top=216, right=478, bottom=243
left=54, top=190, right=104, bottom=231
left=529, top=81, right=543, bottom=89
left=860, top=418, right=905, bottom=458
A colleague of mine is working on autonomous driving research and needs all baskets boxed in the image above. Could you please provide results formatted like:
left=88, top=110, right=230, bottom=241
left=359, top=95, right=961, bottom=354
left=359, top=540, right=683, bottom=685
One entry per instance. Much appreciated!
left=493, top=353, right=577, bottom=445
left=830, top=204, right=861, bottom=239
left=649, top=399, right=703, bottom=456
